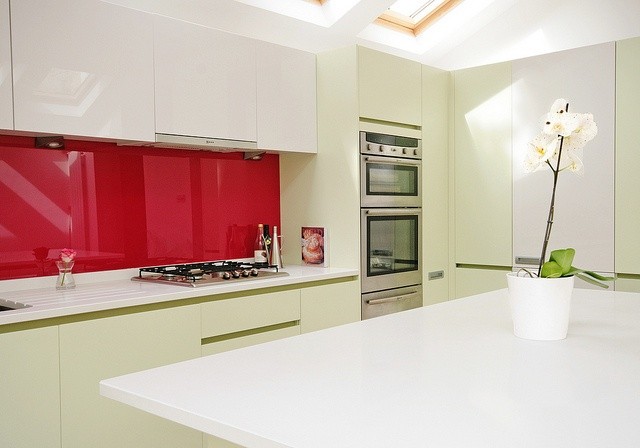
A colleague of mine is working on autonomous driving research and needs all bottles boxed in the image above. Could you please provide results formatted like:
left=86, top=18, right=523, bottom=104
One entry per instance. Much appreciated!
left=264, top=225, right=270, bottom=255
left=254, top=223, right=269, bottom=267
left=271, top=225, right=282, bottom=268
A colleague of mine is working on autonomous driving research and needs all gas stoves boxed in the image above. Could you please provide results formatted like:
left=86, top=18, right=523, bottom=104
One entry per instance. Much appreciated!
left=131, top=261, right=290, bottom=287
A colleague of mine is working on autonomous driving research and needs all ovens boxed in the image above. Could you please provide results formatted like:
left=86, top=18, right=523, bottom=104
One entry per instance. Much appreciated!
left=360, top=120, right=422, bottom=319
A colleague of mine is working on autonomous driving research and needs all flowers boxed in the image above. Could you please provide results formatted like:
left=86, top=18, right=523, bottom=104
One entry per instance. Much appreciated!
left=527, top=96, right=598, bottom=277
left=60, top=248, right=78, bottom=262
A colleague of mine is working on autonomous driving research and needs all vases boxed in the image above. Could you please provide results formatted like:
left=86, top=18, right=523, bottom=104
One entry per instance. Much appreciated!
left=56, top=261, right=76, bottom=289
left=506, top=270, right=575, bottom=343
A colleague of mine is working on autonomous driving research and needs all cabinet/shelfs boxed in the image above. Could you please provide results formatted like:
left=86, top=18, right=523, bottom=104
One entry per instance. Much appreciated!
left=59, top=304, right=202, bottom=448
left=254, top=40, right=319, bottom=156
left=202, top=289, right=301, bottom=357
left=300, top=278, right=361, bottom=333
left=0, top=318, right=62, bottom=448
left=10, top=0, right=154, bottom=146
left=0, top=0, right=14, bottom=133
left=154, top=10, right=256, bottom=149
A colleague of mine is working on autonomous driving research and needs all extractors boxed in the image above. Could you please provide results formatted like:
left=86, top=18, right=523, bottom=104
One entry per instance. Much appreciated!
left=155, top=133, right=258, bottom=148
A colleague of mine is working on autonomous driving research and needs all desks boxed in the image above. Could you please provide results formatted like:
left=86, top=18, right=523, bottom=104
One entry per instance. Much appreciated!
left=97, top=282, right=639, bottom=447
